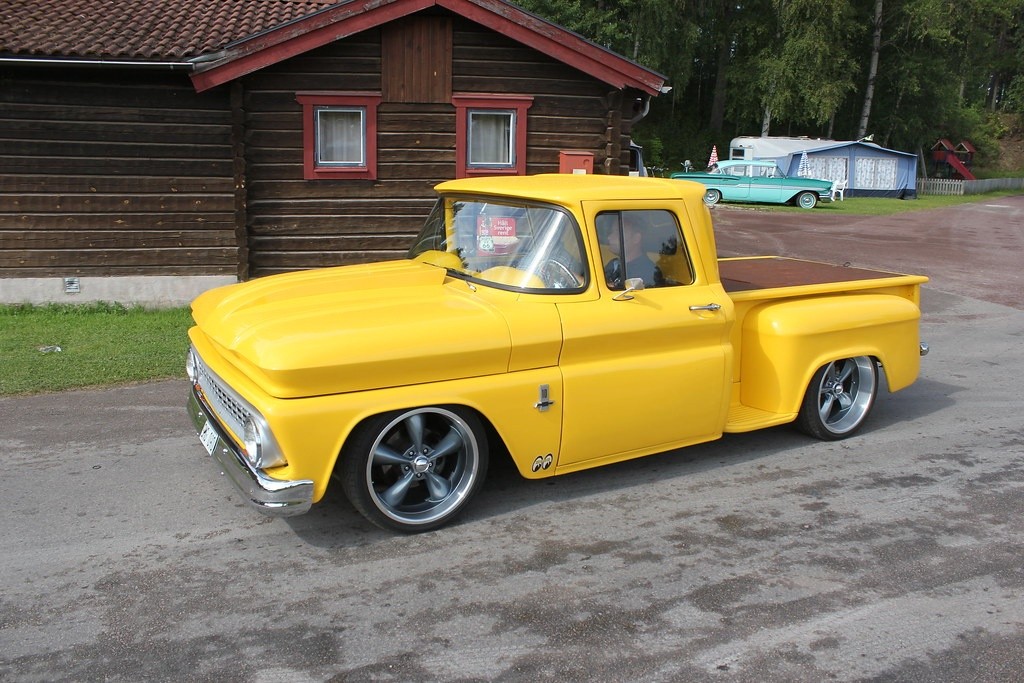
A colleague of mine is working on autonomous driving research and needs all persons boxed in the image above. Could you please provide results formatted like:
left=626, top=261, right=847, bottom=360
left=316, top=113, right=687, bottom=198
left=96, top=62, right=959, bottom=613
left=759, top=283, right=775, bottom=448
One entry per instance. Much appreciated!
left=559, top=214, right=664, bottom=291
left=514, top=211, right=579, bottom=288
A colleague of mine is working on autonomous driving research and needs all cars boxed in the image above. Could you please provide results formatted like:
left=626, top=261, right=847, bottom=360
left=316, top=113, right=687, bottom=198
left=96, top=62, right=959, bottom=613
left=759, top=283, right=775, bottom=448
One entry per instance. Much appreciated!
left=670, top=159, right=833, bottom=210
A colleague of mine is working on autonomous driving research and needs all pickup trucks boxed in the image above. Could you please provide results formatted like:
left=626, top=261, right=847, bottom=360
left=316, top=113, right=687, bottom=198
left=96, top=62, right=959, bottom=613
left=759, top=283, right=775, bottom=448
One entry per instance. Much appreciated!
left=186, top=174, right=930, bottom=535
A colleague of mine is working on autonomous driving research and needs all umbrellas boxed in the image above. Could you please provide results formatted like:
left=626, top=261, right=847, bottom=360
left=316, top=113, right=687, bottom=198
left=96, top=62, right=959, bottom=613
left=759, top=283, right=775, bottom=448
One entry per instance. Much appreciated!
left=707, top=145, right=718, bottom=168
left=798, top=149, right=811, bottom=178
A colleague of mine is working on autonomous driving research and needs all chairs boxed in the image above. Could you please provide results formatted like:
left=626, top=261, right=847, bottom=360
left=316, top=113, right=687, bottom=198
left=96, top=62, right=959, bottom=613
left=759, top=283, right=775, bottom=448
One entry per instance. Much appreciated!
left=830, top=178, right=848, bottom=201
